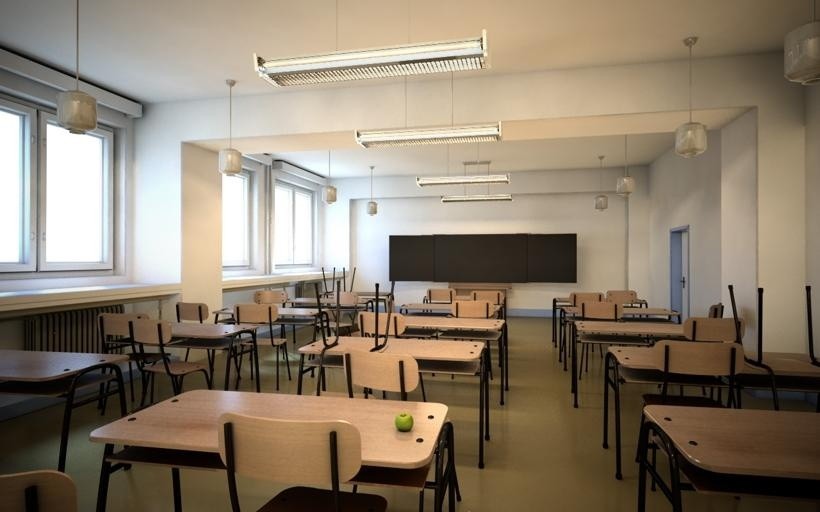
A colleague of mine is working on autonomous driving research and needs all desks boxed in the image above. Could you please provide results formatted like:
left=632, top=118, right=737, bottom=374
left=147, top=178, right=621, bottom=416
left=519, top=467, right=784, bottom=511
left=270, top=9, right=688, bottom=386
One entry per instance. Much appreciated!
left=288, top=297, right=373, bottom=336
left=319, top=290, right=395, bottom=313
left=570, top=319, right=693, bottom=409
left=90, top=389, right=463, bottom=512
left=553, top=297, right=648, bottom=355
left=601, top=343, right=820, bottom=479
left=379, top=315, right=510, bottom=406
left=106, top=319, right=262, bottom=394
left=632, top=403, right=820, bottom=512
left=400, top=302, right=502, bottom=368
left=297, top=336, right=492, bottom=470
left=0, top=348, right=131, bottom=509
left=254, top=290, right=292, bottom=349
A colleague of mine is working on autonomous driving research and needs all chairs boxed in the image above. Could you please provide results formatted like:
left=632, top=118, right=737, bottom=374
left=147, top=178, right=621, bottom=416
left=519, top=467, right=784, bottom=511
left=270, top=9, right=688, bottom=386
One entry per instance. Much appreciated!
left=313, top=279, right=396, bottom=401
left=572, top=291, right=606, bottom=311
left=577, top=301, right=623, bottom=375
left=685, top=316, right=746, bottom=343
left=424, top=288, right=457, bottom=307
left=636, top=340, right=744, bottom=489
left=234, top=304, right=291, bottom=390
left=318, top=289, right=358, bottom=338
left=471, top=290, right=504, bottom=306
left=173, top=302, right=242, bottom=389
left=355, top=310, right=427, bottom=402
left=216, top=410, right=388, bottom=509
left=97, top=311, right=172, bottom=413
left=358, top=312, right=404, bottom=339
left=449, top=302, right=495, bottom=382
left=727, top=283, right=820, bottom=412
left=0, top=469, right=76, bottom=512
left=605, top=290, right=637, bottom=306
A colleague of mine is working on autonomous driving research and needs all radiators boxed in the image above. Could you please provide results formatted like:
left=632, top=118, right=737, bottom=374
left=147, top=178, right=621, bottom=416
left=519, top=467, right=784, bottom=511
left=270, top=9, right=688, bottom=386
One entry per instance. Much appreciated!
left=295, top=284, right=322, bottom=296
left=21, top=305, right=125, bottom=355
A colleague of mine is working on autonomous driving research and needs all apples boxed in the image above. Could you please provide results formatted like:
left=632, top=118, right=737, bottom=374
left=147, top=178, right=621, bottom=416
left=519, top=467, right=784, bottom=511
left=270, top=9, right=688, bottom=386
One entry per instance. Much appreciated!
left=395, top=412, right=413, bottom=432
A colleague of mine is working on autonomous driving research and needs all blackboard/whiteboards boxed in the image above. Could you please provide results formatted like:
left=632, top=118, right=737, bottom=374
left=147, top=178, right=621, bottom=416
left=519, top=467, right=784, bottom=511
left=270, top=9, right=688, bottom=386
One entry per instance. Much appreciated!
left=389, top=233, right=577, bottom=283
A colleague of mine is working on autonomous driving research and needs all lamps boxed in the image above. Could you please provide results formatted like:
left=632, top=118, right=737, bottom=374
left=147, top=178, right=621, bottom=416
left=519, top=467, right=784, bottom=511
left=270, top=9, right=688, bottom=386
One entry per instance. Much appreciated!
left=782, top=21, right=818, bottom=88
left=414, top=144, right=511, bottom=188
left=355, top=73, right=502, bottom=148
left=250, top=2, right=490, bottom=88
left=323, top=149, right=337, bottom=205
left=365, top=169, right=377, bottom=215
left=219, top=80, right=242, bottom=175
left=595, top=155, right=610, bottom=210
left=675, top=37, right=711, bottom=158
left=52, top=1, right=97, bottom=133
left=440, top=163, right=512, bottom=204
left=615, top=136, right=636, bottom=195
